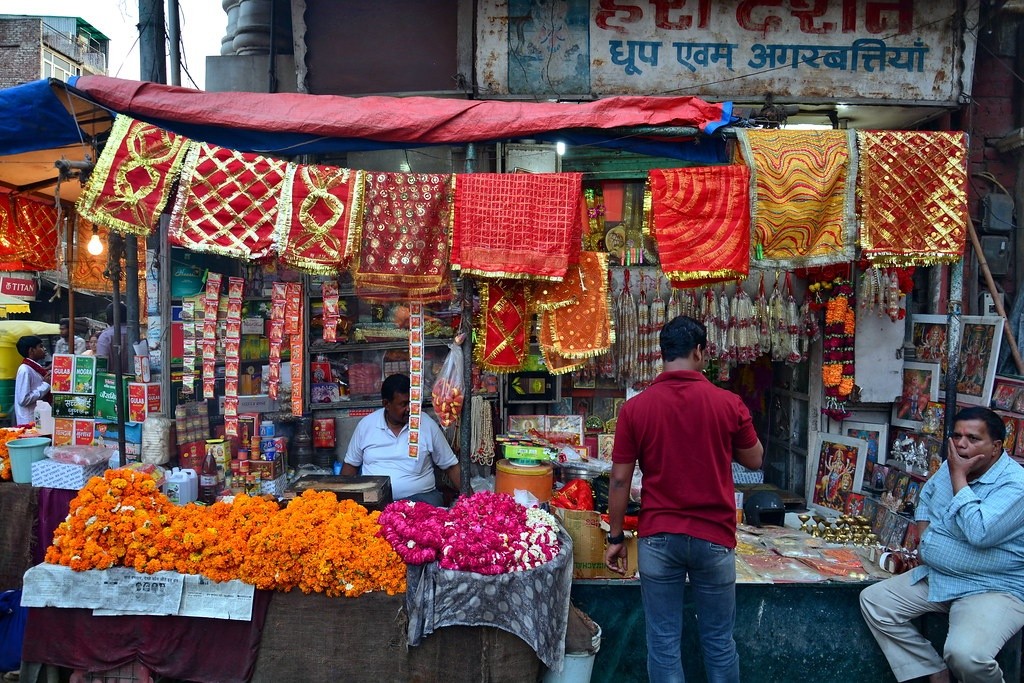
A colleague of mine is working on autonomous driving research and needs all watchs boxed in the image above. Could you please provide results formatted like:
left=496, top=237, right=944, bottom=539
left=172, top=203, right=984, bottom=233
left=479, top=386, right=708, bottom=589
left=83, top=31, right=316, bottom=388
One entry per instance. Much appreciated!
left=607, top=532, right=624, bottom=544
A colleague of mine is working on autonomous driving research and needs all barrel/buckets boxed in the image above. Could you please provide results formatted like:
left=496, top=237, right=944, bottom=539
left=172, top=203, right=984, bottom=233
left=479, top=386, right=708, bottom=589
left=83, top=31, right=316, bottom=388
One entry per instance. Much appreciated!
left=316, top=448, right=339, bottom=470
left=541, top=619, right=602, bottom=683
left=5, top=437, right=51, bottom=485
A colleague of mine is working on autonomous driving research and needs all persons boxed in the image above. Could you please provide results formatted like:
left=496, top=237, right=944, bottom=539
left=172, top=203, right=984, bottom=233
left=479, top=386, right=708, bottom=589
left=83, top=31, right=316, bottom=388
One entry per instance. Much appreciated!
left=81, top=330, right=101, bottom=356
left=338, top=373, right=459, bottom=507
left=859, top=407, right=1024, bottom=683
left=44, top=319, right=84, bottom=371
left=97, top=302, right=128, bottom=373
left=13, top=336, right=52, bottom=424
left=605, top=317, right=764, bottom=683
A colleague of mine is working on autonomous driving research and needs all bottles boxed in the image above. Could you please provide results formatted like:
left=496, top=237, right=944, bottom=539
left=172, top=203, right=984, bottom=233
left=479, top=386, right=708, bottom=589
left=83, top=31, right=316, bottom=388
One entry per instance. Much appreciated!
left=225, top=470, right=262, bottom=498
left=163, top=470, right=191, bottom=505
left=200, top=448, right=220, bottom=505
left=172, top=467, right=199, bottom=502
